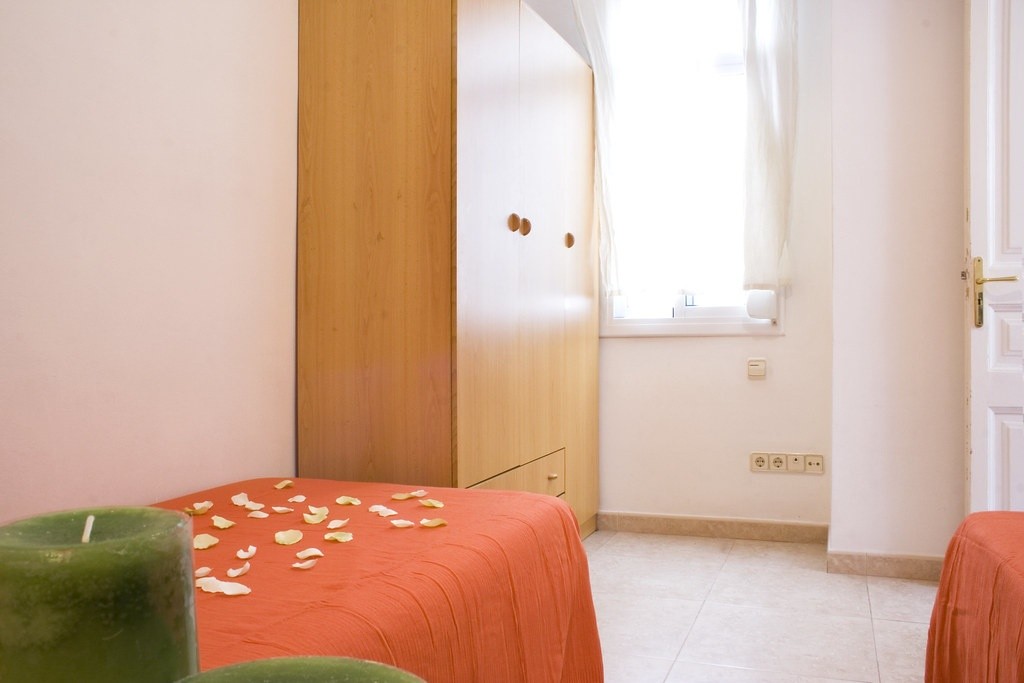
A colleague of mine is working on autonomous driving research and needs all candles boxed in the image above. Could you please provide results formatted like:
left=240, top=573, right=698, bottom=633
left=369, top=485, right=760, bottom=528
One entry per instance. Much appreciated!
left=0, top=506, right=199, bottom=683
left=176, top=656, right=427, bottom=683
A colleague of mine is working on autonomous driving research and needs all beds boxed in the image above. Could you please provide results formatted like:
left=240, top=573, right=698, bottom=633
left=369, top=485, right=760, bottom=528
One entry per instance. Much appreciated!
left=924, top=510, right=1023, bottom=683
left=144, top=478, right=605, bottom=683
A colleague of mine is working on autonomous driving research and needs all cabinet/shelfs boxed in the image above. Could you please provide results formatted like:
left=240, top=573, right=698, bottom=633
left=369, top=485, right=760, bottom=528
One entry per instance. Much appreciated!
left=294, top=0, right=600, bottom=542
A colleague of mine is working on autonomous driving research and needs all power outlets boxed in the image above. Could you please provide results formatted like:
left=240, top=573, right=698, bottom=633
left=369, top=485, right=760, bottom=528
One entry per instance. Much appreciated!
left=750, top=453, right=787, bottom=472
